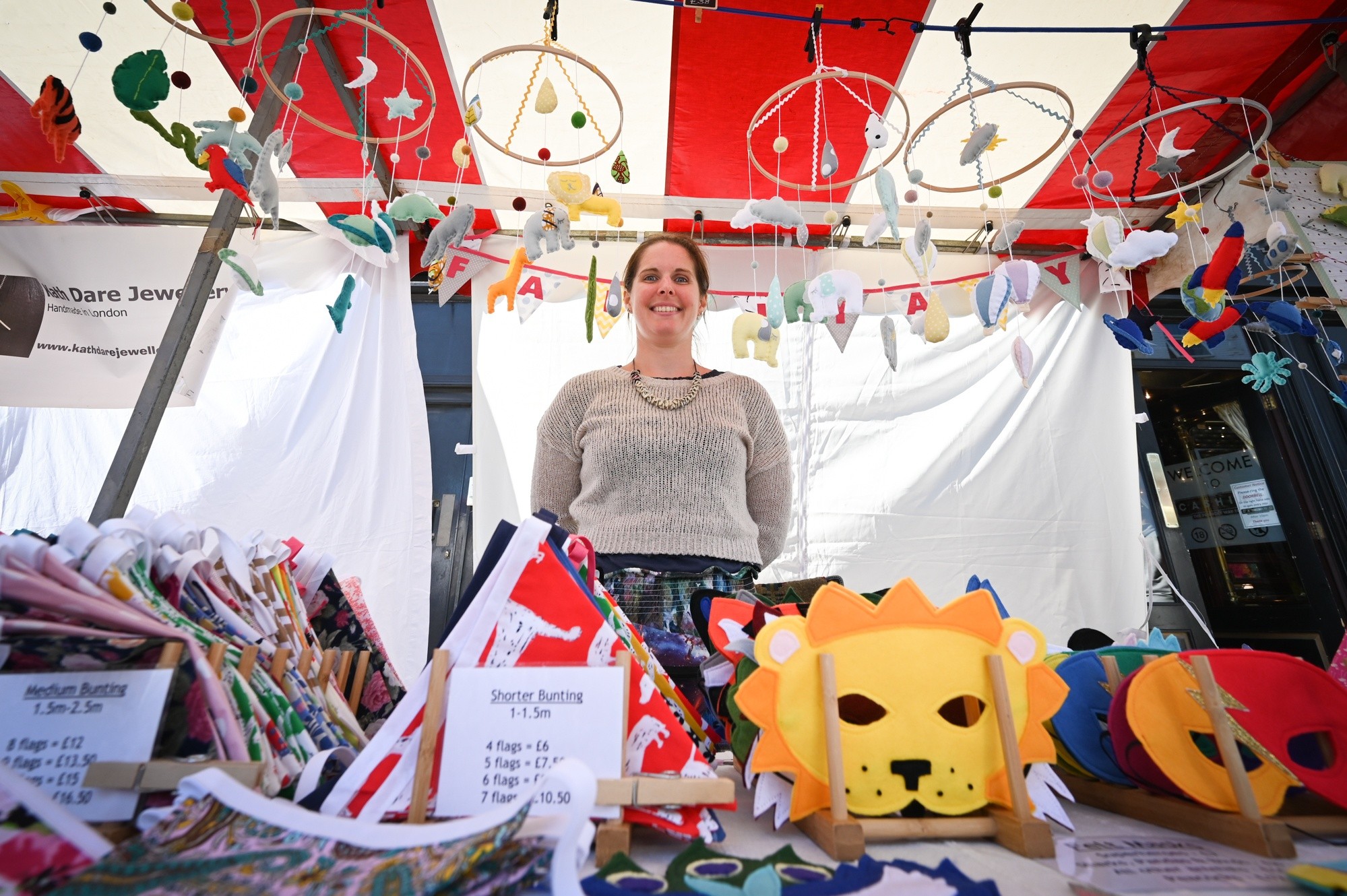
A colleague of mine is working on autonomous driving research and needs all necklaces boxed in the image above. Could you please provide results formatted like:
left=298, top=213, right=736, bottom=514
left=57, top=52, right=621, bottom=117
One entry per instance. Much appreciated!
left=630, top=357, right=702, bottom=409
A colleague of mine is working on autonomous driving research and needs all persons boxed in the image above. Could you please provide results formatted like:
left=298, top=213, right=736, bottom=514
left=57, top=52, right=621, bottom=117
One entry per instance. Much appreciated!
left=531, top=231, right=792, bottom=643
